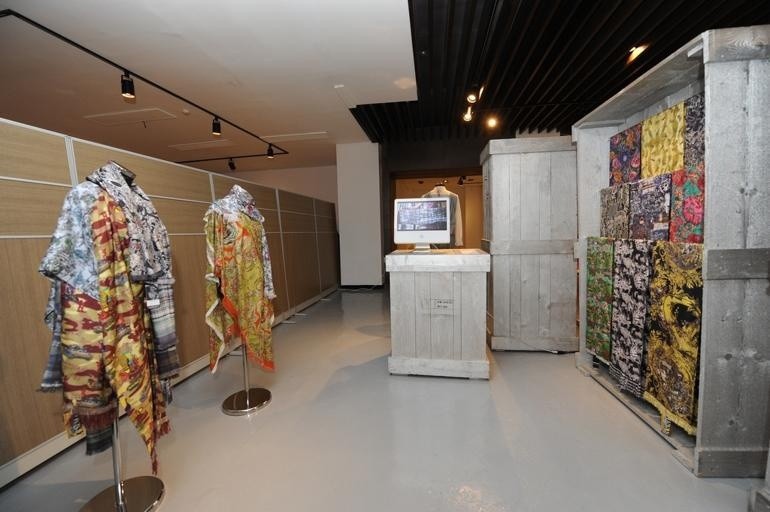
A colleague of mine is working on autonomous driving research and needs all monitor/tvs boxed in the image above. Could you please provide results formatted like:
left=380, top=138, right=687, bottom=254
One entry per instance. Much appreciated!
left=394, top=197, right=450, bottom=253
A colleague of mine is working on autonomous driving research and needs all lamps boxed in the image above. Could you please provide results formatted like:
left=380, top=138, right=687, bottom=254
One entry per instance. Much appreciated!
left=465, top=80, right=478, bottom=104
left=457, top=176, right=467, bottom=185
left=228, top=158, right=236, bottom=169
left=211, top=115, right=221, bottom=136
left=120, top=69, right=136, bottom=99
left=266, top=142, right=273, bottom=159
left=464, top=104, right=472, bottom=122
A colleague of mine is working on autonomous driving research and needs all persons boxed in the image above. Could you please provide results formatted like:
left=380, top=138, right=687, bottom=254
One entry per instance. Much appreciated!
left=202, top=182, right=279, bottom=373
left=421, top=184, right=464, bottom=249
left=39, top=161, right=176, bottom=473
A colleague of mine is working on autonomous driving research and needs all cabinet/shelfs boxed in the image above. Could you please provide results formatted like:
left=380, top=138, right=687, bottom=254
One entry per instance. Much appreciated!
left=572, top=25, right=768, bottom=477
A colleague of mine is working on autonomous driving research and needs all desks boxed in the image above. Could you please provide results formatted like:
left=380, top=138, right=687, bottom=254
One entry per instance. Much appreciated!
left=385, top=247, right=496, bottom=379
